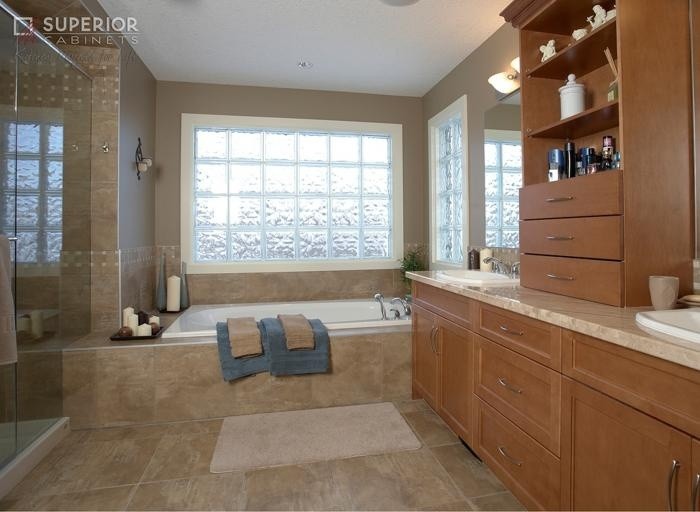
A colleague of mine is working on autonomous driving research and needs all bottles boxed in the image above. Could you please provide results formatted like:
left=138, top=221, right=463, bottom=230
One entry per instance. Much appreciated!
left=549, top=136, right=614, bottom=183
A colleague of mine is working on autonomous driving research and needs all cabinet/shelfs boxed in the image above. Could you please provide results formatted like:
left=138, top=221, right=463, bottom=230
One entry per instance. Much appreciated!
left=411, top=280, right=473, bottom=451
left=499, top=0, right=695, bottom=310
left=473, top=300, right=562, bottom=511
left=563, top=328, right=700, bottom=511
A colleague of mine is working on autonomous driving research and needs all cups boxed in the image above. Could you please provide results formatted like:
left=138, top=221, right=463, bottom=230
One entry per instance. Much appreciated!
left=558, top=73, right=586, bottom=121
left=648, top=275, right=679, bottom=312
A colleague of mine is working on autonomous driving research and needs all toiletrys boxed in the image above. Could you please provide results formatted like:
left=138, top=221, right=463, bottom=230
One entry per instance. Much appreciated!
left=547, top=135, right=621, bottom=182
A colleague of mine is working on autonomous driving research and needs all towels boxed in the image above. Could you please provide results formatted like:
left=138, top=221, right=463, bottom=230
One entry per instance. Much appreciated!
left=0, top=234, right=18, bottom=365
left=215, top=313, right=330, bottom=382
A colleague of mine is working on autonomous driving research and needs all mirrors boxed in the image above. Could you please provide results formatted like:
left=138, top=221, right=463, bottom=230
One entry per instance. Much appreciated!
left=483, top=88, right=522, bottom=250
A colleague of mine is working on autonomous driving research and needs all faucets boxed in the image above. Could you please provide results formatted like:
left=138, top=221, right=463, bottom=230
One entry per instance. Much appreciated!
left=374, top=293, right=387, bottom=320
left=389, top=297, right=411, bottom=315
left=484, top=257, right=509, bottom=274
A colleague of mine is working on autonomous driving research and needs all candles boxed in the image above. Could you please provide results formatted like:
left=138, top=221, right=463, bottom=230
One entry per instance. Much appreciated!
left=16, top=309, right=45, bottom=338
left=123, top=306, right=160, bottom=336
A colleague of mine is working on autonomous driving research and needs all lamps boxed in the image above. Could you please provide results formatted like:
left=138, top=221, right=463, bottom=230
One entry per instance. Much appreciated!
left=488, top=56, right=520, bottom=93
left=135, top=137, right=152, bottom=181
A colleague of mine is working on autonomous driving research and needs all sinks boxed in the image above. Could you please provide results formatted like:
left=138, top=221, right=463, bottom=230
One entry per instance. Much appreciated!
left=637, top=306, right=696, bottom=341
left=439, top=268, right=519, bottom=284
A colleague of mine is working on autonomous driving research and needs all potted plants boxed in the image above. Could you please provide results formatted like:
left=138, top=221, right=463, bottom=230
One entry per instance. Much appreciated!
left=398, top=249, right=425, bottom=301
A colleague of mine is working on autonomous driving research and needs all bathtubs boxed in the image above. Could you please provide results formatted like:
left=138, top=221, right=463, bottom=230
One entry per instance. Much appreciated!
left=162, top=299, right=414, bottom=337
left=18, top=308, right=60, bottom=336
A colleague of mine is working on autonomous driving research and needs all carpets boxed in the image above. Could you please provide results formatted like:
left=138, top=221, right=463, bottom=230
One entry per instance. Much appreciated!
left=209, top=401, right=421, bottom=474
